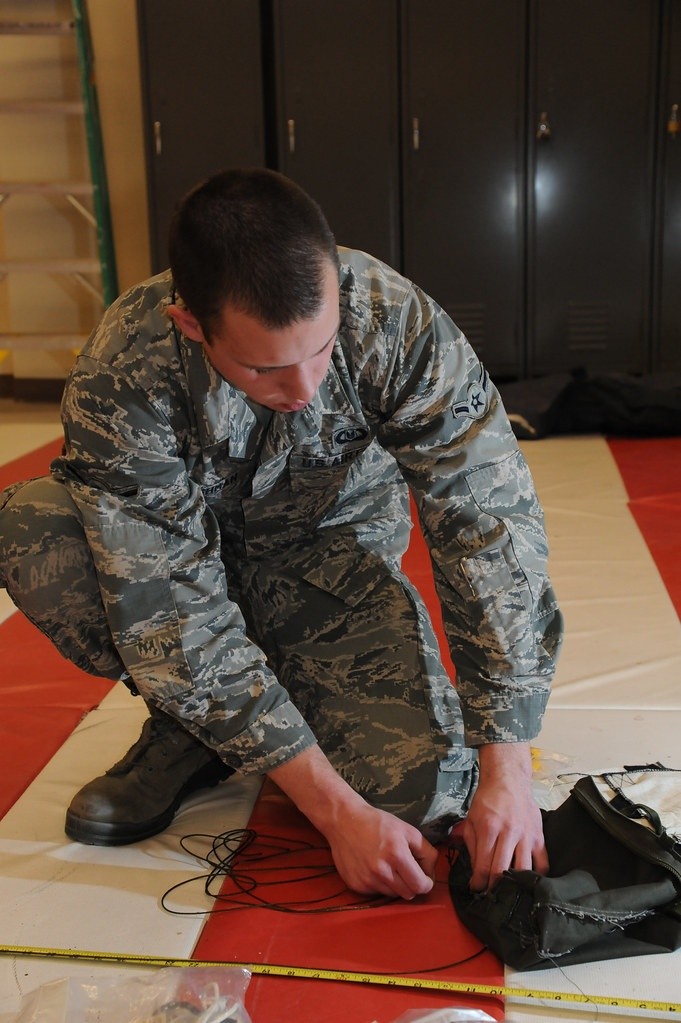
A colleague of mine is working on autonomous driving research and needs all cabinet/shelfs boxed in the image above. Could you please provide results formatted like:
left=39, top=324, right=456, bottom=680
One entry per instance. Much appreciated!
left=0, top=0, right=119, bottom=354
left=138, top=0, right=681, bottom=381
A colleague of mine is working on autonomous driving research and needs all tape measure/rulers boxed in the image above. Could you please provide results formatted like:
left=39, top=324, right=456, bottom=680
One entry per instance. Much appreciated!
left=0, top=941, right=680, bottom=1022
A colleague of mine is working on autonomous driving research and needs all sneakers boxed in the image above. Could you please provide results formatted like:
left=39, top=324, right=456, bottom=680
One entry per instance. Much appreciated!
left=64, top=697, right=237, bottom=847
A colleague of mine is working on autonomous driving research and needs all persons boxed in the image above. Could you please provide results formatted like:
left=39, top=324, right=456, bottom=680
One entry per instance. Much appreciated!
left=0, top=165, right=564, bottom=898
